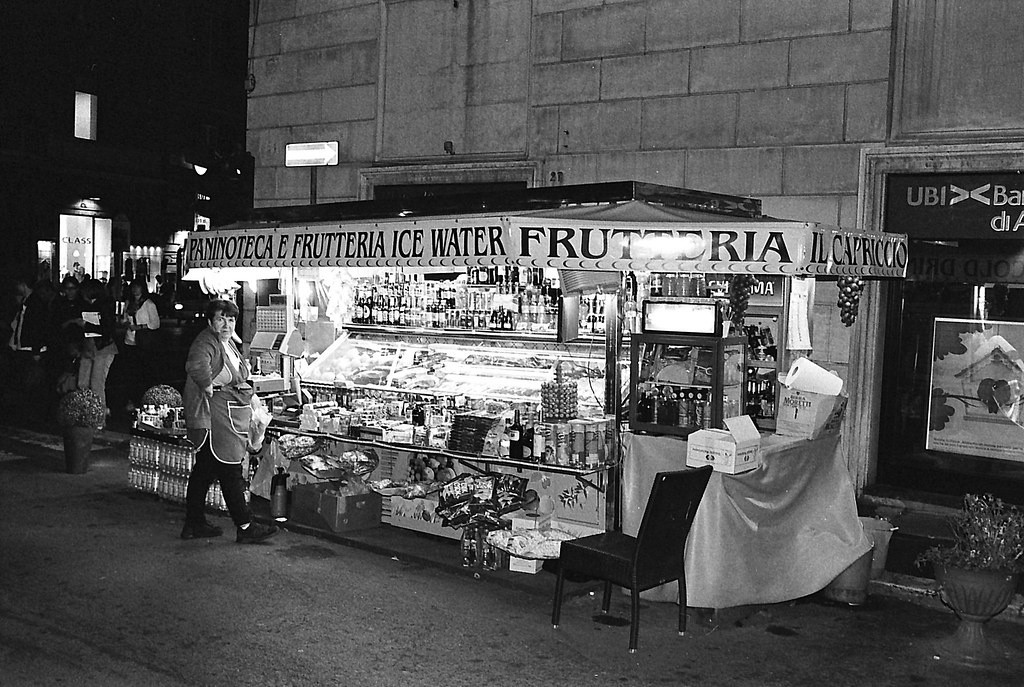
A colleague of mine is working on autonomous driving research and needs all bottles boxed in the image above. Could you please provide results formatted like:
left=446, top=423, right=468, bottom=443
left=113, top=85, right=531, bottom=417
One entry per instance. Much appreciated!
left=412, top=402, right=424, bottom=426
left=747, top=379, right=774, bottom=419
left=204, top=480, right=227, bottom=506
left=623, top=287, right=649, bottom=335
left=651, top=273, right=706, bottom=297
left=244, top=357, right=263, bottom=377
left=620, top=270, right=648, bottom=297
left=396, top=391, right=458, bottom=412
left=510, top=409, right=524, bottom=460
left=125, top=435, right=192, bottom=499
left=466, top=265, right=558, bottom=332
left=301, top=384, right=389, bottom=407
left=192, top=448, right=201, bottom=466
left=578, top=293, right=605, bottom=334
left=131, top=403, right=168, bottom=429
left=351, top=271, right=466, bottom=329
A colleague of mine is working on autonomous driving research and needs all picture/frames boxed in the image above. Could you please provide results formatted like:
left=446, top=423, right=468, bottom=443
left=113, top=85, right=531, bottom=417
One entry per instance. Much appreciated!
left=925, top=316, right=1024, bottom=462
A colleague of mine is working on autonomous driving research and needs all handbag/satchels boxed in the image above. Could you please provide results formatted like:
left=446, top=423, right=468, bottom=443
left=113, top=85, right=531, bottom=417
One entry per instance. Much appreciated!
left=135, top=327, right=162, bottom=348
left=218, top=383, right=255, bottom=404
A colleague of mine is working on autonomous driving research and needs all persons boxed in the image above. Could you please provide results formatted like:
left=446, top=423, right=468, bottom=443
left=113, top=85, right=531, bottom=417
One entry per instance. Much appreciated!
left=1, top=262, right=174, bottom=432
left=182, top=300, right=280, bottom=542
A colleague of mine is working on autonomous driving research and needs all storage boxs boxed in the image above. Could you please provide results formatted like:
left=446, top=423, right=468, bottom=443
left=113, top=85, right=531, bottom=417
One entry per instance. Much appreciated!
left=303, top=322, right=644, bottom=551
left=288, top=483, right=382, bottom=533
left=509, top=512, right=551, bottom=575
left=685, top=414, right=761, bottom=475
left=775, top=356, right=848, bottom=441
left=629, top=332, right=749, bottom=441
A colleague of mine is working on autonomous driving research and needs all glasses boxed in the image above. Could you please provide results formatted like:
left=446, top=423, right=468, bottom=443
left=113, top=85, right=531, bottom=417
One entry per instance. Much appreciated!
left=63, top=286, right=76, bottom=290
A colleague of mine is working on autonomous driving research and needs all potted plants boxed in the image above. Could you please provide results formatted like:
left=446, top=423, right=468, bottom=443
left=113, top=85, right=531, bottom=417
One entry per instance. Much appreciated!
left=61, top=389, right=104, bottom=474
left=914, top=494, right=1024, bottom=670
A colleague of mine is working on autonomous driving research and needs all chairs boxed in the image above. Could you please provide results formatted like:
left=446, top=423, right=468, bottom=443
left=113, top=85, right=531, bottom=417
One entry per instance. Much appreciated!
left=551, top=464, right=714, bottom=655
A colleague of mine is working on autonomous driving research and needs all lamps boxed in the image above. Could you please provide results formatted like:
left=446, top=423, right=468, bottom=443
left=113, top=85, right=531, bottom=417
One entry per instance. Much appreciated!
left=642, top=297, right=732, bottom=336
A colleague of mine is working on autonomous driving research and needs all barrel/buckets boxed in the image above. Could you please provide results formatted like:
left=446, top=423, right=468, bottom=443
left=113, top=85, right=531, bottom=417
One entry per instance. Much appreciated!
left=854, top=517, right=894, bottom=579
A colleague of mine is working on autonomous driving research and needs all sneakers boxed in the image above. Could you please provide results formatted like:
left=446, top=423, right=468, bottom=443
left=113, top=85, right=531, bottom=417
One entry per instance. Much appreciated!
left=236, top=523, right=279, bottom=543
left=180, top=521, right=223, bottom=539
